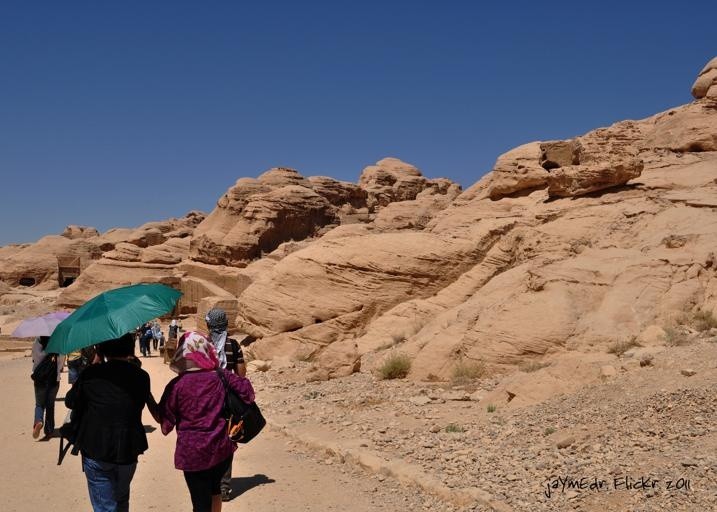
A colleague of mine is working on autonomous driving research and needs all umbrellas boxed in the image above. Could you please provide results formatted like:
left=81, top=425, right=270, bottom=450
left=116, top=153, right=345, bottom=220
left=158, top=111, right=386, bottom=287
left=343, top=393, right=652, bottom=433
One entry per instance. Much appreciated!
left=43, top=284, right=184, bottom=354
left=10, top=311, right=71, bottom=338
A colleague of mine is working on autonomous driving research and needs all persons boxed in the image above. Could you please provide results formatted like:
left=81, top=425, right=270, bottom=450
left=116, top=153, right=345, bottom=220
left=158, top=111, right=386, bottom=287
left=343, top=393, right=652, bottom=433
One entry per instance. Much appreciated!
left=205, top=308, right=247, bottom=501
left=64, top=332, right=150, bottom=512
left=137, top=318, right=182, bottom=357
left=145, top=332, right=255, bottom=512
left=29, top=336, right=62, bottom=439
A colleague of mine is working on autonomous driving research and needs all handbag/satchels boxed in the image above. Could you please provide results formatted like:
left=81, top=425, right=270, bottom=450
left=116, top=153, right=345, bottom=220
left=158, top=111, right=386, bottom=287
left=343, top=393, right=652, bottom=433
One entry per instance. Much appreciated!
left=214, top=368, right=266, bottom=443
left=31, top=353, right=59, bottom=381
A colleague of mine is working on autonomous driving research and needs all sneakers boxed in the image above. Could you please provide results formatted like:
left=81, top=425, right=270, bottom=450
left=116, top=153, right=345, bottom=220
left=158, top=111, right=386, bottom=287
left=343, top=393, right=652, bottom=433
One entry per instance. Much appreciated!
left=221, top=488, right=231, bottom=501
left=33, top=422, right=43, bottom=438
